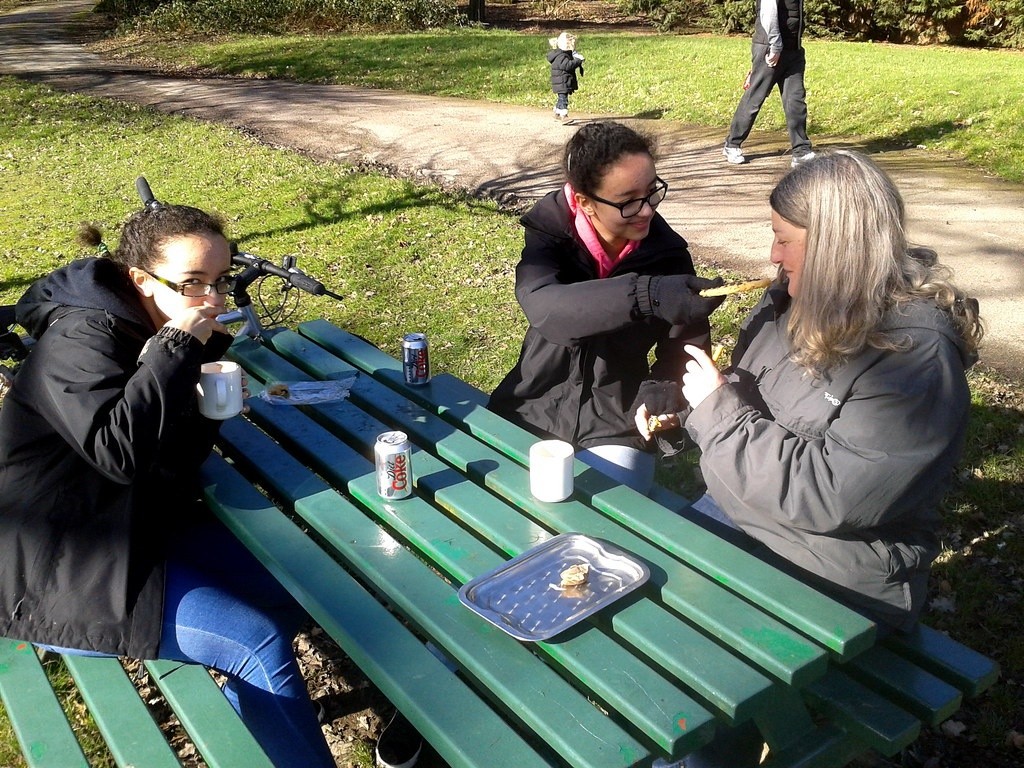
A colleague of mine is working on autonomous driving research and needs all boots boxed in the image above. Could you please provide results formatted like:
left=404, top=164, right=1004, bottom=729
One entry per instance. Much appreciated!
left=559, top=109, right=575, bottom=125
left=553, top=106, right=562, bottom=122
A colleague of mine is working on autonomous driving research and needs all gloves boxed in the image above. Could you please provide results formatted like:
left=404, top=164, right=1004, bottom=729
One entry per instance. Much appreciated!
left=627, top=381, right=689, bottom=422
left=649, top=275, right=727, bottom=326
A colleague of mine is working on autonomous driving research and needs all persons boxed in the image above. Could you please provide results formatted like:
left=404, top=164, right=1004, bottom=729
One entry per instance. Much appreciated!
left=374, top=119, right=731, bottom=768
left=722, top=0, right=817, bottom=168
left=546, top=32, right=586, bottom=125
left=0, top=200, right=339, bottom=768
left=631, top=149, right=987, bottom=768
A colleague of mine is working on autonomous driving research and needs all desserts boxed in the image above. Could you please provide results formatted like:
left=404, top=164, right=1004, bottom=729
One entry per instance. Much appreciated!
left=270, top=384, right=290, bottom=398
left=559, top=563, right=590, bottom=586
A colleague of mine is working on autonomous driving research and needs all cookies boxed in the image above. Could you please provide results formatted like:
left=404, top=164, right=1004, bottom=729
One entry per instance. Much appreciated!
left=648, top=415, right=662, bottom=431
left=698, top=277, right=771, bottom=297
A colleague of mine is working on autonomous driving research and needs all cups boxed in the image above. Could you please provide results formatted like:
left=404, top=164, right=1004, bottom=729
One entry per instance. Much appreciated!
left=529, top=439, right=575, bottom=503
left=197, top=360, right=244, bottom=421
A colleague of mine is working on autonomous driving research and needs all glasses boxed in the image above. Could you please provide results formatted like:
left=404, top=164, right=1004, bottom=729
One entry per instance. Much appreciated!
left=142, top=267, right=238, bottom=297
left=589, top=176, right=668, bottom=219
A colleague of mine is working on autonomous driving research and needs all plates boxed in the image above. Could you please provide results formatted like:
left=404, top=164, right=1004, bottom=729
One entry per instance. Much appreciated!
left=458, top=531, right=651, bottom=642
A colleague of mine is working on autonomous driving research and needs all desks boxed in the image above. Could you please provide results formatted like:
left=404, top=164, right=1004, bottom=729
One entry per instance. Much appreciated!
left=189, top=318, right=878, bottom=768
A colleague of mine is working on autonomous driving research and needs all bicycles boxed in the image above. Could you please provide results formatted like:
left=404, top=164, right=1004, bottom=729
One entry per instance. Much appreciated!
left=0, top=176, right=345, bottom=391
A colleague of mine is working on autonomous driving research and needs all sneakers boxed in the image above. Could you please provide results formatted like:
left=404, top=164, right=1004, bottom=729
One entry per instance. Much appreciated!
left=791, top=152, right=816, bottom=168
left=722, top=142, right=744, bottom=164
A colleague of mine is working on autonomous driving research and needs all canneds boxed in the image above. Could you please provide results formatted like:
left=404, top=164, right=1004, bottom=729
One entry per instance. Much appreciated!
left=402, top=333, right=432, bottom=386
left=373, top=430, right=413, bottom=501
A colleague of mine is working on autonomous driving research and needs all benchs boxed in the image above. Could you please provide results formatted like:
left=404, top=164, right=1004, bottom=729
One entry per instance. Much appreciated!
left=0, top=639, right=269, bottom=768
left=431, top=373, right=1002, bottom=754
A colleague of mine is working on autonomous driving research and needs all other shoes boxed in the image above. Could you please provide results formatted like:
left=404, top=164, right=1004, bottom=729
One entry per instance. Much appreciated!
left=376, top=709, right=422, bottom=768
left=652, top=753, right=712, bottom=768
left=312, top=699, right=325, bottom=722
